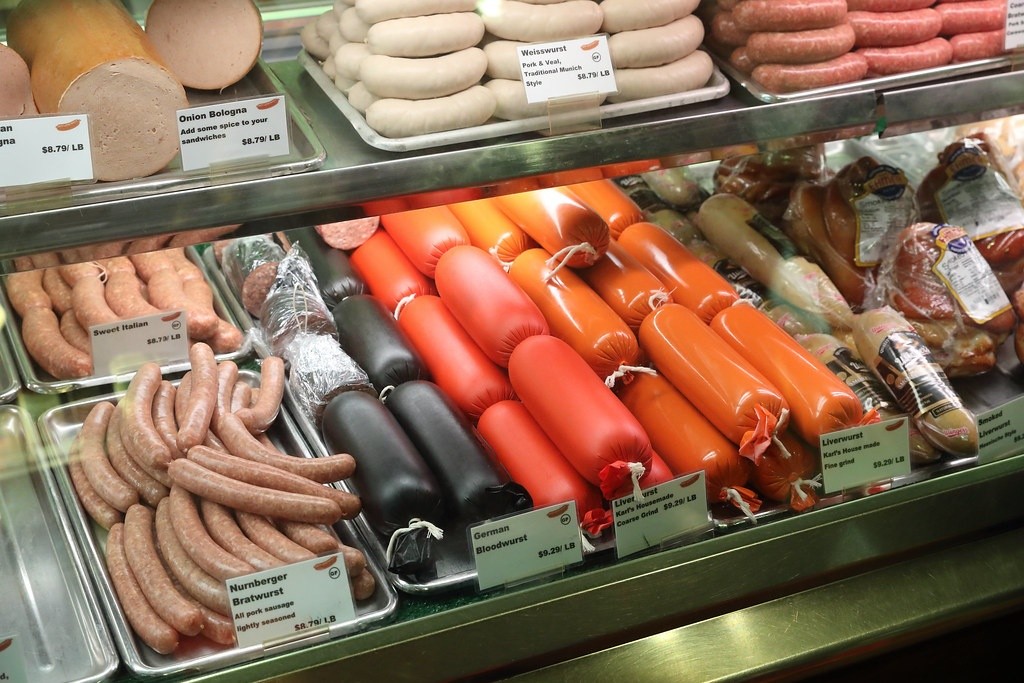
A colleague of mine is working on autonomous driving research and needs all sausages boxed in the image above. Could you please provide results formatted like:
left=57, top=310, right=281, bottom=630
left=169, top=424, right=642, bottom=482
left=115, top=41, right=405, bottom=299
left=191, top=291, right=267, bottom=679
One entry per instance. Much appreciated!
left=2, top=125, right=981, bottom=664
left=0, top=0, right=1024, bottom=183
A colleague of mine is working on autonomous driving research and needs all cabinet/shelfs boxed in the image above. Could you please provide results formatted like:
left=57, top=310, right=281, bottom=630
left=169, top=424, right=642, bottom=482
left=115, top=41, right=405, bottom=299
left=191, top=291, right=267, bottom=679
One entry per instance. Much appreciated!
left=0, top=19, right=1024, bottom=683
